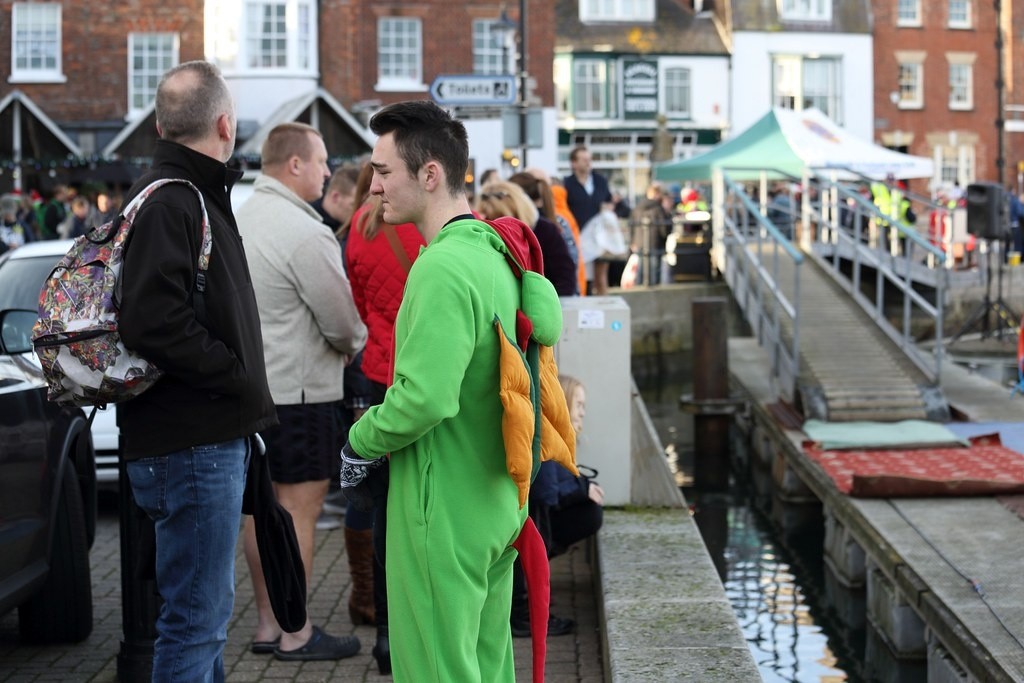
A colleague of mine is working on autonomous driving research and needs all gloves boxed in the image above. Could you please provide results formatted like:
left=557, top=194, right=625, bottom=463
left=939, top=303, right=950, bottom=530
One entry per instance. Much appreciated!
left=338, top=447, right=387, bottom=509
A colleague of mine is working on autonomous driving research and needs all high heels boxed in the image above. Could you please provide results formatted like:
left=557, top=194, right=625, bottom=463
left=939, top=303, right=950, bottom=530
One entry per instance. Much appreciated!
left=372, top=633, right=393, bottom=676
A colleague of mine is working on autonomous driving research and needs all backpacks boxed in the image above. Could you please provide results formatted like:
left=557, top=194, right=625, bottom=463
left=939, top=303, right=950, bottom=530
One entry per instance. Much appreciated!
left=31, top=178, right=213, bottom=407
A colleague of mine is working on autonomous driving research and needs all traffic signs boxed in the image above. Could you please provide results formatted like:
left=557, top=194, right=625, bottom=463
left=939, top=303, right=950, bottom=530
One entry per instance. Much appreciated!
left=430, top=74, right=521, bottom=108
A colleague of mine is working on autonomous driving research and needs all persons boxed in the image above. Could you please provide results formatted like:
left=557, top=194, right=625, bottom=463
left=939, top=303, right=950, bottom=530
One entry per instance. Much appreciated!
left=117, top=59, right=282, bottom=683
left=227, top=124, right=708, bottom=674
left=0, top=182, right=131, bottom=253
left=735, top=173, right=916, bottom=259
left=1003, top=184, right=1024, bottom=265
left=339, top=100, right=582, bottom=683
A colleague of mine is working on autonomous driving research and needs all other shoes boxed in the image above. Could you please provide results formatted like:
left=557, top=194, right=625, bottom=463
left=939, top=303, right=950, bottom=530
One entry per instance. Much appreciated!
left=511, top=599, right=575, bottom=635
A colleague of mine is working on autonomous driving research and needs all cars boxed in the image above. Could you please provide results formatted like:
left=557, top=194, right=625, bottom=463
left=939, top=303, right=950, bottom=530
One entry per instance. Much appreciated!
left=0, top=239, right=120, bottom=645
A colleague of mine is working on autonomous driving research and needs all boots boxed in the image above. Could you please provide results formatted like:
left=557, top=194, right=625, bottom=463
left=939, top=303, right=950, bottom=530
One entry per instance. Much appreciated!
left=344, top=524, right=376, bottom=624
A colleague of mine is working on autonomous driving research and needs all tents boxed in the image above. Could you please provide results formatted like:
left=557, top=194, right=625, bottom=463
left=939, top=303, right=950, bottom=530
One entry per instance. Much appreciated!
left=654, top=104, right=938, bottom=199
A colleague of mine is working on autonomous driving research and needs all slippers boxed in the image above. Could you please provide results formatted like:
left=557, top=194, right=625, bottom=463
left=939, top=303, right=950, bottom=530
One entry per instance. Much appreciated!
left=255, top=641, right=279, bottom=652
left=273, top=625, right=359, bottom=660
